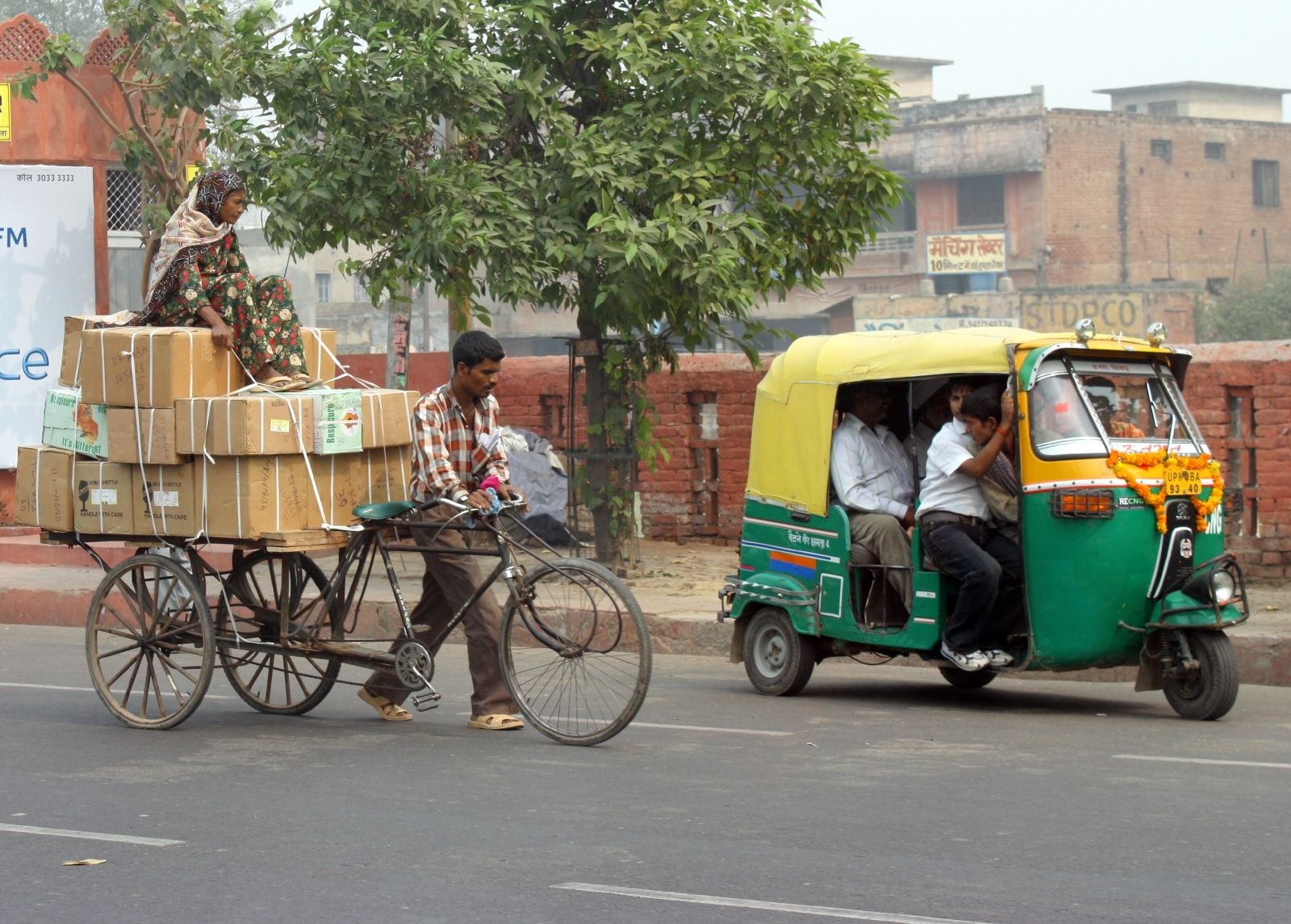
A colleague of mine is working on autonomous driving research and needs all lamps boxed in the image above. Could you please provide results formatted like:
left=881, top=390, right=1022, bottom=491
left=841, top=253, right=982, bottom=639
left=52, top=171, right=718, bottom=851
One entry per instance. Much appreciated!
left=890, top=293, right=901, bottom=301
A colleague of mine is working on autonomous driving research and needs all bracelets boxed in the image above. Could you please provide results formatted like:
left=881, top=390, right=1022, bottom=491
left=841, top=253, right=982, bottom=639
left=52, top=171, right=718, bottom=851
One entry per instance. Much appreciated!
left=997, top=426, right=1008, bottom=437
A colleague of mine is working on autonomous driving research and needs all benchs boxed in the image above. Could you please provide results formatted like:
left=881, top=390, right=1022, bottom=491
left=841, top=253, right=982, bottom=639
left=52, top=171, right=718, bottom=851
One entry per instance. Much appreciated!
left=832, top=502, right=877, bottom=567
left=911, top=498, right=947, bottom=575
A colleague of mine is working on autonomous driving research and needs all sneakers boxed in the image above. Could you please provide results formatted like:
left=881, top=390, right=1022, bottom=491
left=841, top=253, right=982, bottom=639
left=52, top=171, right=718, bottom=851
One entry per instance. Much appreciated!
left=940, top=641, right=991, bottom=672
left=982, top=649, right=1015, bottom=667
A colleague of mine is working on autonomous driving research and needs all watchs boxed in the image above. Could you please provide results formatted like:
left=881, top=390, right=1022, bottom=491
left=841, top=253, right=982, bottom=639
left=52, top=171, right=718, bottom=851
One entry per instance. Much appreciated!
left=457, top=495, right=469, bottom=505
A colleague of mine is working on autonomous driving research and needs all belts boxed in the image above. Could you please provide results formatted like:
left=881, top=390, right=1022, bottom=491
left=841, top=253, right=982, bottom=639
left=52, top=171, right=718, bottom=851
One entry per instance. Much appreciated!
left=920, top=512, right=979, bottom=526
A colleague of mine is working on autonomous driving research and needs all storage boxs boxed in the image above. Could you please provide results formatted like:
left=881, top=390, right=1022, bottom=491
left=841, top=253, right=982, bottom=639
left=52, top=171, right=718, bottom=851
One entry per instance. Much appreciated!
left=15, top=314, right=420, bottom=539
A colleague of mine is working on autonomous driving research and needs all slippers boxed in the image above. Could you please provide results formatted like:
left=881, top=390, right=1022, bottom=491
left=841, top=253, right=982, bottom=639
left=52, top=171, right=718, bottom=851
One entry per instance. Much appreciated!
left=292, top=374, right=323, bottom=390
left=357, top=686, right=413, bottom=721
left=467, top=713, right=525, bottom=729
left=249, top=375, right=306, bottom=392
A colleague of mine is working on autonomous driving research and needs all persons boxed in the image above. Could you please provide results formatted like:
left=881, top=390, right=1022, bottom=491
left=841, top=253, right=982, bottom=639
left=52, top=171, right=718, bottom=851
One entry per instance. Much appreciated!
left=149, top=170, right=322, bottom=391
left=831, top=374, right=1145, bottom=671
left=353, top=329, right=527, bottom=728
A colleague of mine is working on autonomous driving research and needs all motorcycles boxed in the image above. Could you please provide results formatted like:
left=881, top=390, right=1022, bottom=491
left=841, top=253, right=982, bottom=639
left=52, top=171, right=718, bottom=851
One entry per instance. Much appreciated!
left=717, top=317, right=1251, bottom=721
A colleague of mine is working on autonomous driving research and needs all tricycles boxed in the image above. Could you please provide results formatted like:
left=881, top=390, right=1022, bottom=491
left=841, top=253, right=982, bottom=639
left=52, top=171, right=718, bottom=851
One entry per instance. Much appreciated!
left=41, top=476, right=653, bottom=745
